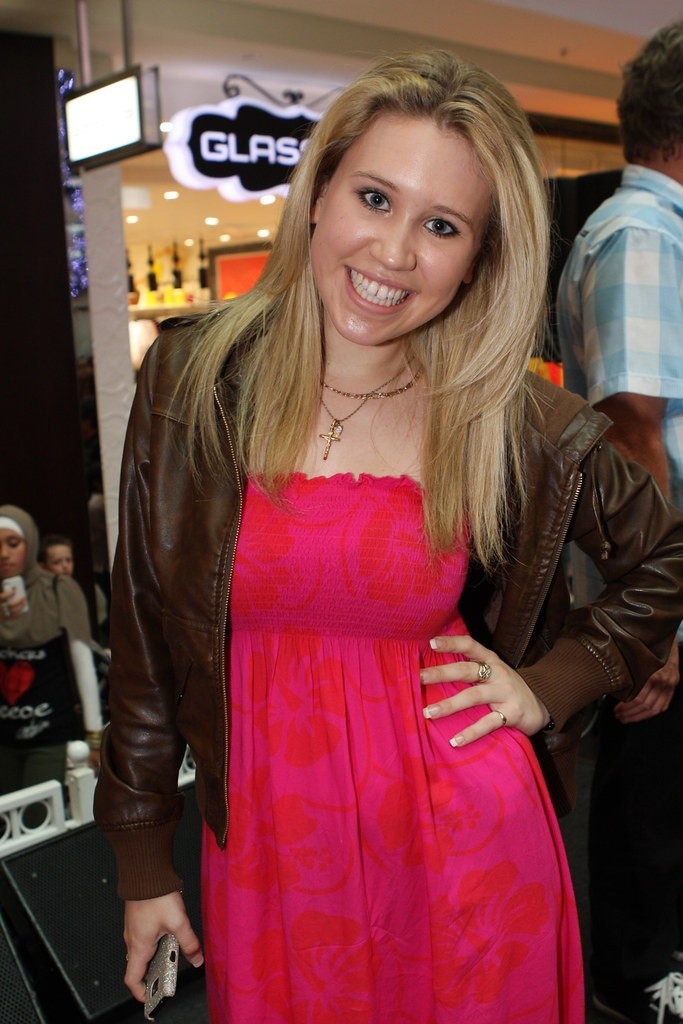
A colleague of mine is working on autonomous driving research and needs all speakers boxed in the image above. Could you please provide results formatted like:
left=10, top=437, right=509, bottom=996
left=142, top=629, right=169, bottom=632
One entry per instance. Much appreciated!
left=0, top=781, right=208, bottom=1024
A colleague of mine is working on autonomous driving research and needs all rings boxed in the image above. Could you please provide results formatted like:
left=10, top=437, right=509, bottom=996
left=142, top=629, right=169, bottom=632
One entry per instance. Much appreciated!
left=477, top=662, right=492, bottom=681
left=125, top=955, right=130, bottom=960
left=3, top=602, right=12, bottom=620
left=495, top=710, right=508, bottom=726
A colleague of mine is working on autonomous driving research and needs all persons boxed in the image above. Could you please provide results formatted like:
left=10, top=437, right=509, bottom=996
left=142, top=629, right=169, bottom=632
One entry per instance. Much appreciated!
left=94, top=49, right=683, bottom=1024
left=0, top=504, right=111, bottom=827
left=556, top=20, right=683, bottom=1023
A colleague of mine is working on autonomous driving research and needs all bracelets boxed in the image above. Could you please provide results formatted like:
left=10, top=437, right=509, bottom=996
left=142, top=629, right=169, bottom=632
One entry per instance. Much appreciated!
left=86, top=730, right=107, bottom=753
left=542, top=716, right=557, bottom=733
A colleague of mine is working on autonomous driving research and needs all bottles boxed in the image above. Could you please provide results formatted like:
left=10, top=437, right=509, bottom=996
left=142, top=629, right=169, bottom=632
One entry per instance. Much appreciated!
left=147, top=247, right=157, bottom=290
left=198, top=240, right=210, bottom=287
left=128, top=260, right=134, bottom=292
left=172, top=244, right=182, bottom=289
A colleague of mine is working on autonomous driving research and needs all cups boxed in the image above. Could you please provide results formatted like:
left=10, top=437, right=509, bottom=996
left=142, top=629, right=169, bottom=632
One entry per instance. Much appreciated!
left=3, top=576, right=28, bottom=614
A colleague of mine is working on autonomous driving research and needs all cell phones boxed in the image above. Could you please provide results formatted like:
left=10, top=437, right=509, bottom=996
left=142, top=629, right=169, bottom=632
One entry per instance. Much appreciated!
left=143, top=933, right=178, bottom=1019
left=3, top=576, right=29, bottom=617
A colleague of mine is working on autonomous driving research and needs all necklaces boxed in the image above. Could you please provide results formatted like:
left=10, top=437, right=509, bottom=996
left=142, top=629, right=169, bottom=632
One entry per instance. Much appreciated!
left=315, top=352, right=425, bottom=460
